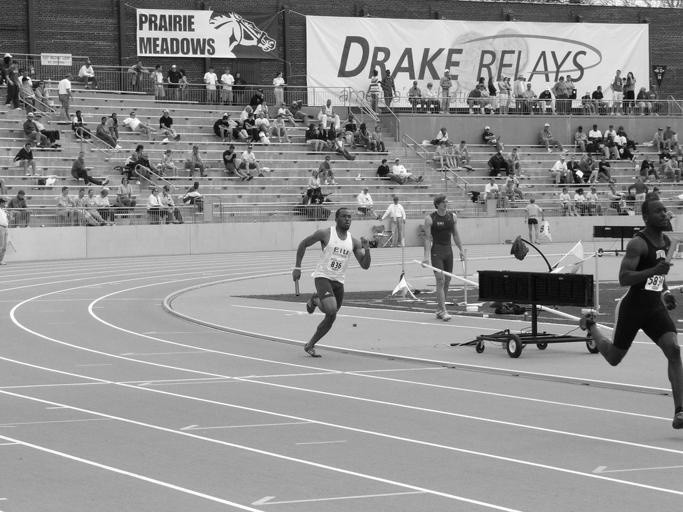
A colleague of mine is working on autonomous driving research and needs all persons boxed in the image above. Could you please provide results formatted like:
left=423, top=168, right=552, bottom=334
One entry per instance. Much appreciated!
left=0, top=52, right=96, bottom=120
left=292, top=207, right=371, bottom=358
left=524, top=199, right=544, bottom=245
left=579, top=198, right=683, bottom=428
left=204, top=66, right=219, bottom=102
left=421, top=194, right=465, bottom=320
left=0, top=200, right=9, bottom=266
left=381, top=197, right=406, bottom=247
left=8, top=109, right=208, bottom=227
left=407, top=68, right=682, bottom=216
left=129, top=60, right=189, bottom=100
left=214, top=71, right=423, bottom=219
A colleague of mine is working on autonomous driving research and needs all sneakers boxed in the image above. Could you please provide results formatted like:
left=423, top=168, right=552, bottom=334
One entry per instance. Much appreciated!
left=305, top=344, right=321, bottom=357
left=436, top=309, right=452, bottom=321
left=307, top=294, right=316, bottom=313
left=673, top=412, right=683, bottom=428
left=580, top=312, right=597, bottom=330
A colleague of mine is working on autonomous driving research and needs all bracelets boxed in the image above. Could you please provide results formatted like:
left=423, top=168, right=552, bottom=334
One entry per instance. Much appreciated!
left=660, top=289, right=671, bottom=298
left=294, top=266, right=303, bottom=270
